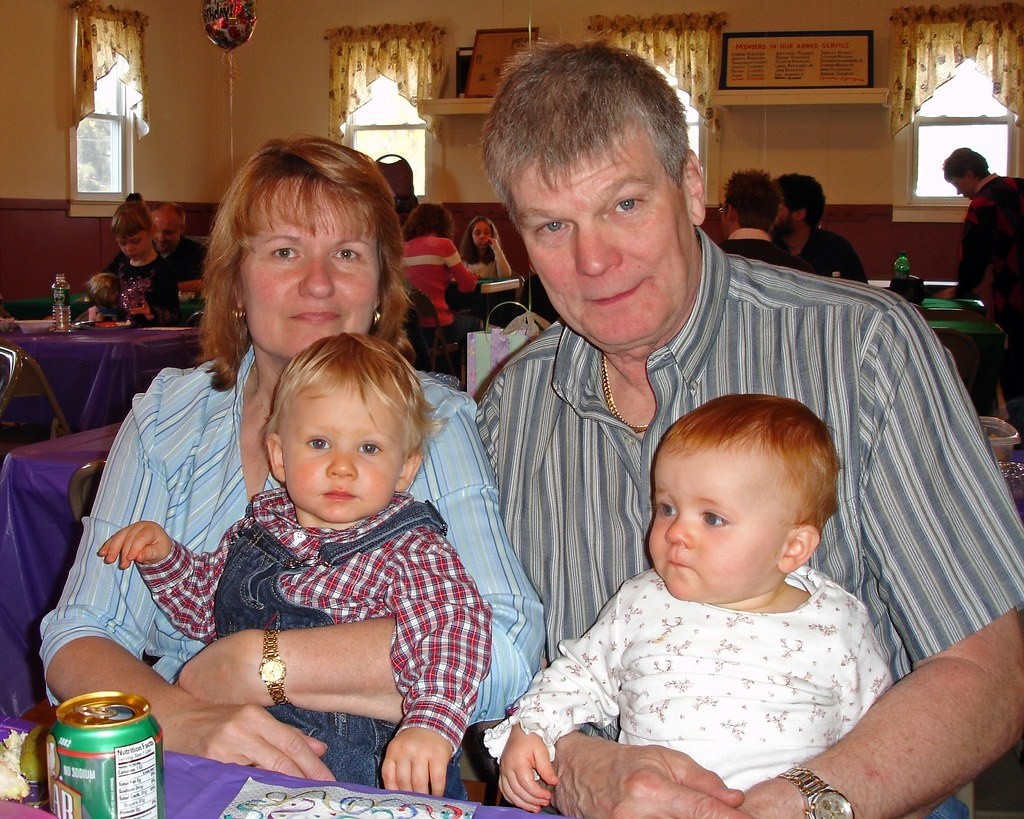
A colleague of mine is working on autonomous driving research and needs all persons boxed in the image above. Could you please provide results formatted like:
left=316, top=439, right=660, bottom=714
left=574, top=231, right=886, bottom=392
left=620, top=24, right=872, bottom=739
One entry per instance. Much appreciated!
left=388, top=202, right=513, bottom=328
left=97, top=332, right=491, bottom=797
left=475, top=35, right=1024, bottom=819
left=716, top=168, right=782, bottom=269
left=934, top=148, right=1024, bottom=463
left=484, top=392, right=894, bottom=814
left=81, top=191, right=210, bottom=325
left=773, top=172, right=870, bottom=286
left=39, top=133, right=547, bottom=801
left=492, top=66, right=501, bottom=76
left=475, top=54, right=483, bottom=65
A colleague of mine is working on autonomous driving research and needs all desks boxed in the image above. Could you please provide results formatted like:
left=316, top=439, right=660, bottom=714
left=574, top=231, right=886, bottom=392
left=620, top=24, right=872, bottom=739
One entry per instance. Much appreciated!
left=450, top=280, right=521, bottom=298
left=1, top=292, right=205, bottom=327
left=0, top=711, right=576, bottom=819
left=914, top=297, right=987, bottom=314
left=924, top=316, right=1008, bottom=352
left=0, top=421, right=122, bottom=720
left=866, top=278, right=958, bottom=293
left=0, top=321, right=202, bottom=434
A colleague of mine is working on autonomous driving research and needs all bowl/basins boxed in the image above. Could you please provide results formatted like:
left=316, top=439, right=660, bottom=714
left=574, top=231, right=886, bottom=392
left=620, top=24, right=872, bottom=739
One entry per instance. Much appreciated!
left=178, top=294, right=196, bottom=303
left=12, top=320, right=56, bottom=334
left=978, top=416, right=1021, bottom=463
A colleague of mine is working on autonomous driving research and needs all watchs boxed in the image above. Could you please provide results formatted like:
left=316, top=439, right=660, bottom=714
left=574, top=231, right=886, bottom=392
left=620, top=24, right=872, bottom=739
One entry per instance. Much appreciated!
left=259, top=630, right=289, bottom=704
left=776, top=766, right=856, bottom=819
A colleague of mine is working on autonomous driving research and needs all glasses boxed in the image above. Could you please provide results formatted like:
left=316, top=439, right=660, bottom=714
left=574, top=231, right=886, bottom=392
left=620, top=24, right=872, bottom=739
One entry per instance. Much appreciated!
left=719, top=207, right=726, bottom=213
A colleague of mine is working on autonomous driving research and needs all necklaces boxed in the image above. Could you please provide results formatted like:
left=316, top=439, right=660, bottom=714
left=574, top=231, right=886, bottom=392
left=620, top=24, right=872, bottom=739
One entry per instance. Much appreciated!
left=251, top=357, right=274, bottom=424
left=595, top=353, right=655, bottom=435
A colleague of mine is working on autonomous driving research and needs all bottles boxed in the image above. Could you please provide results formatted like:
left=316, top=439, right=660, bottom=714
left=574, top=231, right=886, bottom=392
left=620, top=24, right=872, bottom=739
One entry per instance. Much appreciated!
left=893, top=250, right=910, bottom=278
left=832, top=272, right=841, bottom=279
left=51, top=273, right=71, bottom=333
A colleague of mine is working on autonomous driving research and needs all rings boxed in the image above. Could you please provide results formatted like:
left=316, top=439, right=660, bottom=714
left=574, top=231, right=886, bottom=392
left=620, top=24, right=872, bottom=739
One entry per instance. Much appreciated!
left=246, top=761, right=259, bottom=767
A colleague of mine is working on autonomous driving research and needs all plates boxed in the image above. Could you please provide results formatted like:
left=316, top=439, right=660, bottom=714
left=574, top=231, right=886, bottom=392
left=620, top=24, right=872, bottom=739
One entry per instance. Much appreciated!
left=74, top=325, right=132, bottom=330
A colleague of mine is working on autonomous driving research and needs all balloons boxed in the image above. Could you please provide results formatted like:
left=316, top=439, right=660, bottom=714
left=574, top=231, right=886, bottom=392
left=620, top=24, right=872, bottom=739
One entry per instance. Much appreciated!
left=196, top=0, right=255, bottom=54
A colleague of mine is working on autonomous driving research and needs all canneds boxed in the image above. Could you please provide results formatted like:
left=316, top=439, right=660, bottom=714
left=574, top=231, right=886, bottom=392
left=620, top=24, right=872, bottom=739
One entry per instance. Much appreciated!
left=46, top=691, right=166, bottom=819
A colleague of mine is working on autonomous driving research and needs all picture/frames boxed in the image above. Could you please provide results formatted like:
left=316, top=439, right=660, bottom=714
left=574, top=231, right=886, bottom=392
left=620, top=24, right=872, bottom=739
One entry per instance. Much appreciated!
left=464, top=26, right=539, bottom=97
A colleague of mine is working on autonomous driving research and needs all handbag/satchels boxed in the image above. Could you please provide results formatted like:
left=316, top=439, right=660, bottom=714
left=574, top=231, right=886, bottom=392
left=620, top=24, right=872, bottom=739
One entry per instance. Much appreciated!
left=466, top=301, right=553, bottom=405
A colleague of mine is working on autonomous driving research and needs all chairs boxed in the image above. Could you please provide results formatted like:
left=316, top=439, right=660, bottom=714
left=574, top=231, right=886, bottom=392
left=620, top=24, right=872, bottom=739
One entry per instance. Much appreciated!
left=377, top=154, right=415, bottom=222
left=0, top=339, right=72, bottom=465
left=409, top=288, right=459, bottom=377
